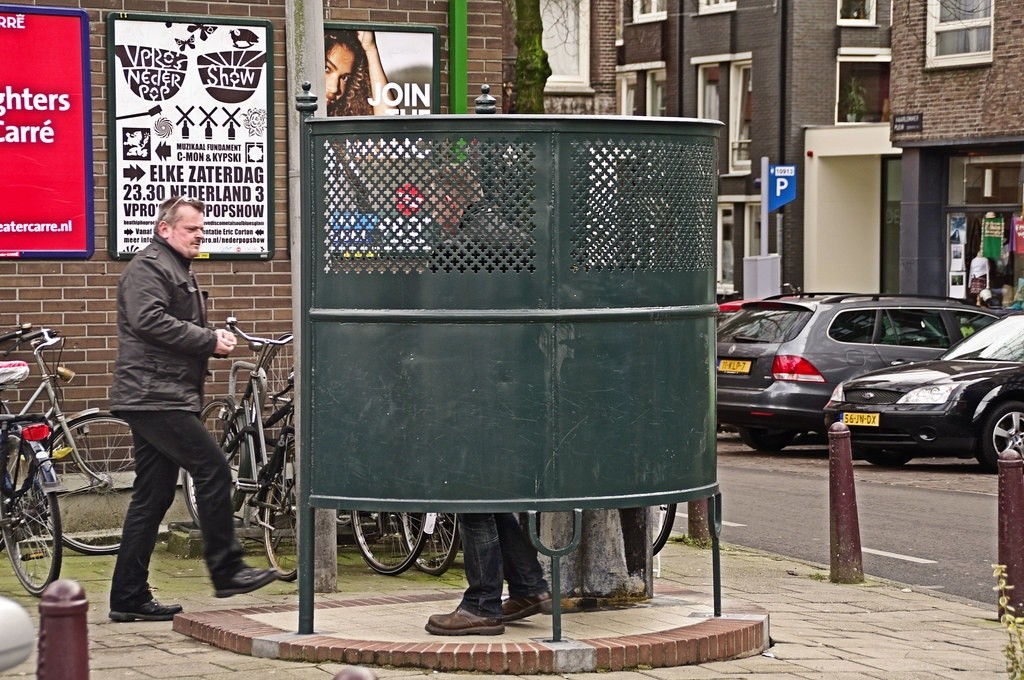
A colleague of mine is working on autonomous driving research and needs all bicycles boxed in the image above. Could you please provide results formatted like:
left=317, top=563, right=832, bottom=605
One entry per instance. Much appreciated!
left=182, top=316, right=678, bottom=582
left=0, top=361, right=62, bottom=596
left=0, top=323, right=136, bottom=555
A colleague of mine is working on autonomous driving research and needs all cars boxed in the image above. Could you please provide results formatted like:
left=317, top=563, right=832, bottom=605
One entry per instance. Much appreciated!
left=821, top=309, right=1024, bottom=471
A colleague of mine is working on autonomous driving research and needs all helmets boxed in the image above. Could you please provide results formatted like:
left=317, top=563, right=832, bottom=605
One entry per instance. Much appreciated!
left=980, top=289, right=992, bottom=301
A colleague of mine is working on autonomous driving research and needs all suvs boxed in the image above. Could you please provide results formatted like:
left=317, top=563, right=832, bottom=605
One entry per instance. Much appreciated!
left=717, top=292, right=1003, bottom=453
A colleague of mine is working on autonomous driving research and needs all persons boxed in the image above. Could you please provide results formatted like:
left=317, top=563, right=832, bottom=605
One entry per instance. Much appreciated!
left=108, top=193, right=281, bottom=622
left=324, top=28, right=401, bottom=212
left=425, top=162, right=553, bottom=635
left=968, top=250, right=990, bottom=305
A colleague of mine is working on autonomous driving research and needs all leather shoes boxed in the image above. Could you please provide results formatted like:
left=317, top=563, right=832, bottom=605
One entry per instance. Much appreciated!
left=108, top=598, right=184, bottom=622
left=425, top=606, right=505, bottom=636
left=214, top=568, right=279, bottom=599
left=501, top=590, right=554, bottom=622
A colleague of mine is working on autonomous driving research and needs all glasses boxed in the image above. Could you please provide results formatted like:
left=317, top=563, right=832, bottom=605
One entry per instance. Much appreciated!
left=160, top=194, right=200, bottom=222
